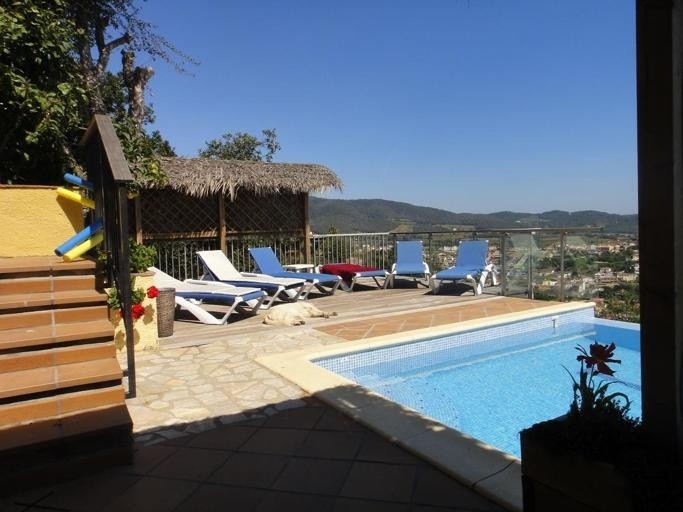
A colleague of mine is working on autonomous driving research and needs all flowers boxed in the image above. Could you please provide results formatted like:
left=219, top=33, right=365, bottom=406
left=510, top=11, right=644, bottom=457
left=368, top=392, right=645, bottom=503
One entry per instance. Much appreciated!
left=108, top=277, right=158, bottom=321
left=567, top=337, right=629, bottom=438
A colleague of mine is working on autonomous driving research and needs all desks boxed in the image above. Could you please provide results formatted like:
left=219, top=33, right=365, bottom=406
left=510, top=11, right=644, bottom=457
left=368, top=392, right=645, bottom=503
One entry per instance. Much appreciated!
left=281, top=262, right=314, bottom=274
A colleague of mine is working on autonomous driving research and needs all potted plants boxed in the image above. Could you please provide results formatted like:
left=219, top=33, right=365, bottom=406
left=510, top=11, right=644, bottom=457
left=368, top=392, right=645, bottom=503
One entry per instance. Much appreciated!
left=128, top=241, right=158, bottom=283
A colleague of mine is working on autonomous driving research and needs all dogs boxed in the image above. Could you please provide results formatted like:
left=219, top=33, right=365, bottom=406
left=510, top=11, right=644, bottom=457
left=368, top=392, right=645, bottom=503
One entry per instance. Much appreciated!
left=262, top=300, right=339, bottom=327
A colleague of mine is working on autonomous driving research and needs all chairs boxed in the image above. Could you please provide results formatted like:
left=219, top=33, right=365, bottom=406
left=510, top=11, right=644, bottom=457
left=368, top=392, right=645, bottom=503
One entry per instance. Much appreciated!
left=387, top=240, right=430, bottom=288
left=148, top=265, right=268, bottom=326
left=315, top=262, right=389, bottom=292
left=244, top=245, right=342, bottom=301
left=196, top=248, right=306, bottom=310
left=428, top=237, right=502, bottom=298
